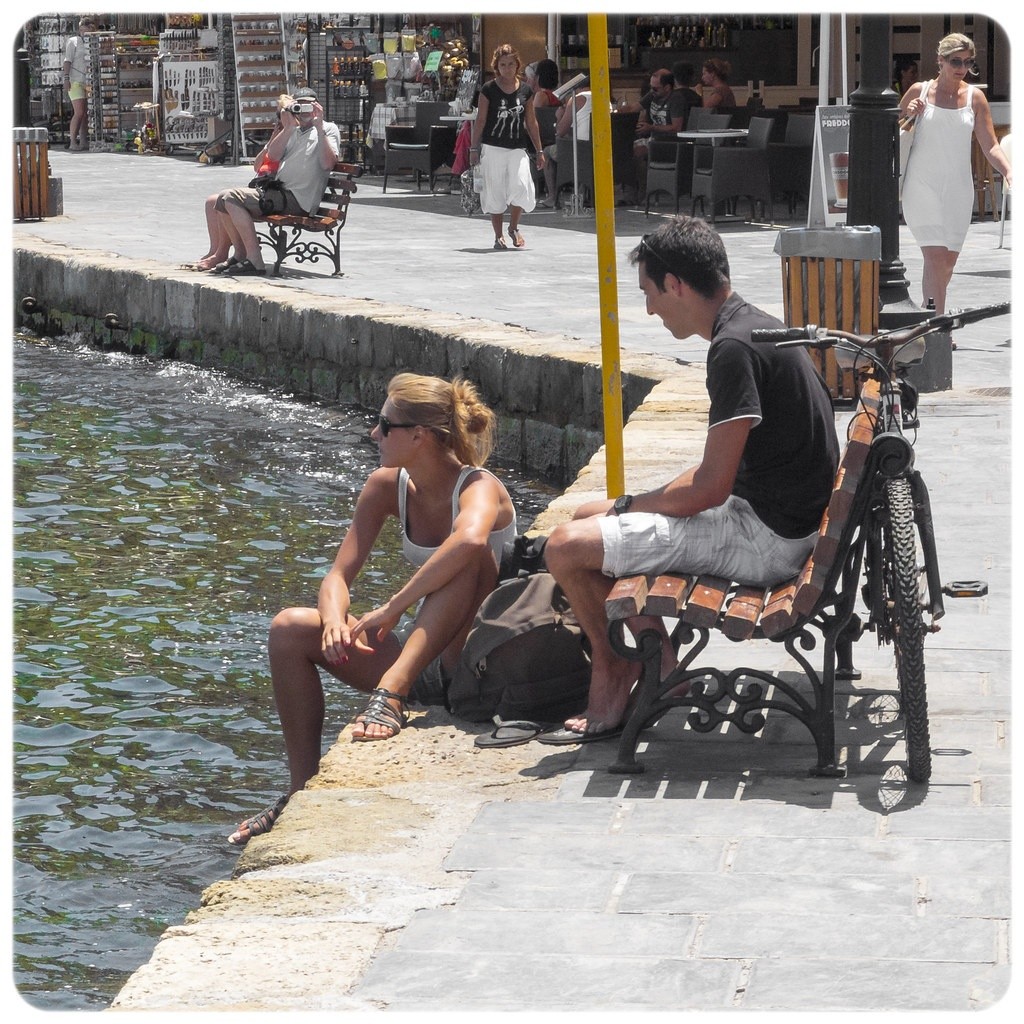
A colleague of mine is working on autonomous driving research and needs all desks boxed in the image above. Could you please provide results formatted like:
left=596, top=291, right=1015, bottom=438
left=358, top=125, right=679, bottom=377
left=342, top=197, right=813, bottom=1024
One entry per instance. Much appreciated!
left=437, top=112, right=484, bottom=164
left=676, top=127, right=751, bottom=155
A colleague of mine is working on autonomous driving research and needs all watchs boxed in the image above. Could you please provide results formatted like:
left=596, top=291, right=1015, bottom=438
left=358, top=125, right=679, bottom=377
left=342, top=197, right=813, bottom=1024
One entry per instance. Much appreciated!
left=614, top=494, right=632, bottom=516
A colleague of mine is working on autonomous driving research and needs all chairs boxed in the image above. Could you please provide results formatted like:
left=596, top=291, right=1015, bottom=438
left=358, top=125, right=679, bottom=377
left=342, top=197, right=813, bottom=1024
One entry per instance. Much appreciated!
left=637, top=96, right=839, bottom=221
left=378, top=100, right=460, bottom=195
left=559, top=113, right=637, bottom=212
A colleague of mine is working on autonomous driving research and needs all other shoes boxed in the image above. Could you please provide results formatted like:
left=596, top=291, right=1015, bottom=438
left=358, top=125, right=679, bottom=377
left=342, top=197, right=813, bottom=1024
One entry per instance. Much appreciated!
left=192, top=252, right=225, bottom=271
left=79, top=140, right=89, bottom=151
left=69, top=143, right=79, bottom=150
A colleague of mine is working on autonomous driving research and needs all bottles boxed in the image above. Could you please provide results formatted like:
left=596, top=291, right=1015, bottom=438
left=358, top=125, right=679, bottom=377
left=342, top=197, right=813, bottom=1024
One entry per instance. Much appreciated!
left=649, top=17, right=726, bottom=46
left=517, top=546, right=539, bottom=576
left=333, top=56, right=369, bottom=96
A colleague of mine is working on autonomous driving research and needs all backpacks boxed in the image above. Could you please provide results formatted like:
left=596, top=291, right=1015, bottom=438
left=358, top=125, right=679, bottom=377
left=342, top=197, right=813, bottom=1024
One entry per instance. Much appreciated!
left=445, top=569, right=593, bottom=724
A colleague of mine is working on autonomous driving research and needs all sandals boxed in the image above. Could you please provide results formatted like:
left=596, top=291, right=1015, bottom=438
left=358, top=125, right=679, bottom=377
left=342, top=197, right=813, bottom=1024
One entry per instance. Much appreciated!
left=351, top=688, right=415, bottom=741
left=508, top=225, right=525, bottom=247
left=227, top=795, right=290, bottom=846
left=494, top=235, right=507, bottom=249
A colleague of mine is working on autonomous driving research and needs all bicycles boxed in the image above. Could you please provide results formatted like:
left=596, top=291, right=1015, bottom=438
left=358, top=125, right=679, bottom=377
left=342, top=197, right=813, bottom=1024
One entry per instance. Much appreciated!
left=752, top=300, right=1011, bottom=787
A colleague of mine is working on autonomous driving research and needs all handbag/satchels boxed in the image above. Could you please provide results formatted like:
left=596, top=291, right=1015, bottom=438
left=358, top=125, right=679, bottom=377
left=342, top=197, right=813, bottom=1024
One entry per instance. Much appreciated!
left=495, top=535, right=549, bottom=583
left=460, top=165, right=482, bottom=217
left=249, top=176, right=287, bottom=215
left=893, top=79, right=934, bottom=201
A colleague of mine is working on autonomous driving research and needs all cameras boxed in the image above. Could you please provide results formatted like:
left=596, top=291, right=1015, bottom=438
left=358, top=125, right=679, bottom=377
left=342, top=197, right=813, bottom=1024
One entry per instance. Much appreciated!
left=292, top=104, right=314, bottom=113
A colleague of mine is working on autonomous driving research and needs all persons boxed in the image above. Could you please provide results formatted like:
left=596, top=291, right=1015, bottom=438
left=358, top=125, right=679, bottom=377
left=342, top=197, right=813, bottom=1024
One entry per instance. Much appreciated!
left=62, top=17, right=97, bottom=150
left=898, top=32, right=1012, bottom=349
left=617, top=68, right=688, bottom=206
left=893, top=55, right=919, bottom=106
left=536, top=217, right=839, bottom=744
left=695, top=57, right=738, bottom=113
left=469, top=44, right=546, bottom=250
left=199, top=87, right=341, bottom=275
left=672, top=64, right=702, bottom=113
left=522, top=58, right=612, bottom=209
left=227, top=373, right=518, bottom=847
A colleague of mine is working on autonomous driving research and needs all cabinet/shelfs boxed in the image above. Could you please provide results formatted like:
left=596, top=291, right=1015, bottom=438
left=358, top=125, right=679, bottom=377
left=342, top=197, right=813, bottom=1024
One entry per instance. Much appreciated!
left=230, top=13, right=296, bottom=163
left=116, top=36, right=160, bottom=140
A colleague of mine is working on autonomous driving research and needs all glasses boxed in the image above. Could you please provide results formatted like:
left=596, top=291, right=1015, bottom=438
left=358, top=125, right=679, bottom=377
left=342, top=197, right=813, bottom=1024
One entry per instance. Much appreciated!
left=276, top=112, right=280, bottom=119
left=943, top=57, right=976, bottom=69
left=649, top=84, right=661, bottom=92
left=378, top=414, right=439, bottom=437
left=638, top=234, right=681, bottom=284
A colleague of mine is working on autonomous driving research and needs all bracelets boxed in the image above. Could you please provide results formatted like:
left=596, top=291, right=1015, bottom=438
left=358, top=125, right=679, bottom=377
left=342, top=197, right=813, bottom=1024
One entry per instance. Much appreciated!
left=470, top=148, right=479, bottom=152
left=64, top=74, right=69, bottom=81
left=536, top=150, right=543, bottom=154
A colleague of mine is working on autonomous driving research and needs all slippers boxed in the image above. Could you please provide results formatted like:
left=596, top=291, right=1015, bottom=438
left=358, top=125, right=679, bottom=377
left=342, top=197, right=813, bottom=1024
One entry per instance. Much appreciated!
left=211, top=256, right=239, bottom=274
left=473, top=713, right=556, bottom=749
left=536, top=200, right=561, bottom=209
left=221, top=259, right=266, bottom=276
left=538, top=722, right=637, bottom=744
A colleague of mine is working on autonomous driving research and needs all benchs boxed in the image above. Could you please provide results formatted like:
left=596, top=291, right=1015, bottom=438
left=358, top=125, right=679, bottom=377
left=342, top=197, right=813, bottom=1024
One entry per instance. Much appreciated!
left=259, top=161, right=364, bottom=274
left=607, top=373, right=881, bottom=784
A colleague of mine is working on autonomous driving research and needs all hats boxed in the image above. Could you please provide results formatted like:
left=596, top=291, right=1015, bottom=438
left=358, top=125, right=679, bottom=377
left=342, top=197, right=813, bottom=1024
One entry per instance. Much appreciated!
left=293, top=86, right=319, bottom=103
